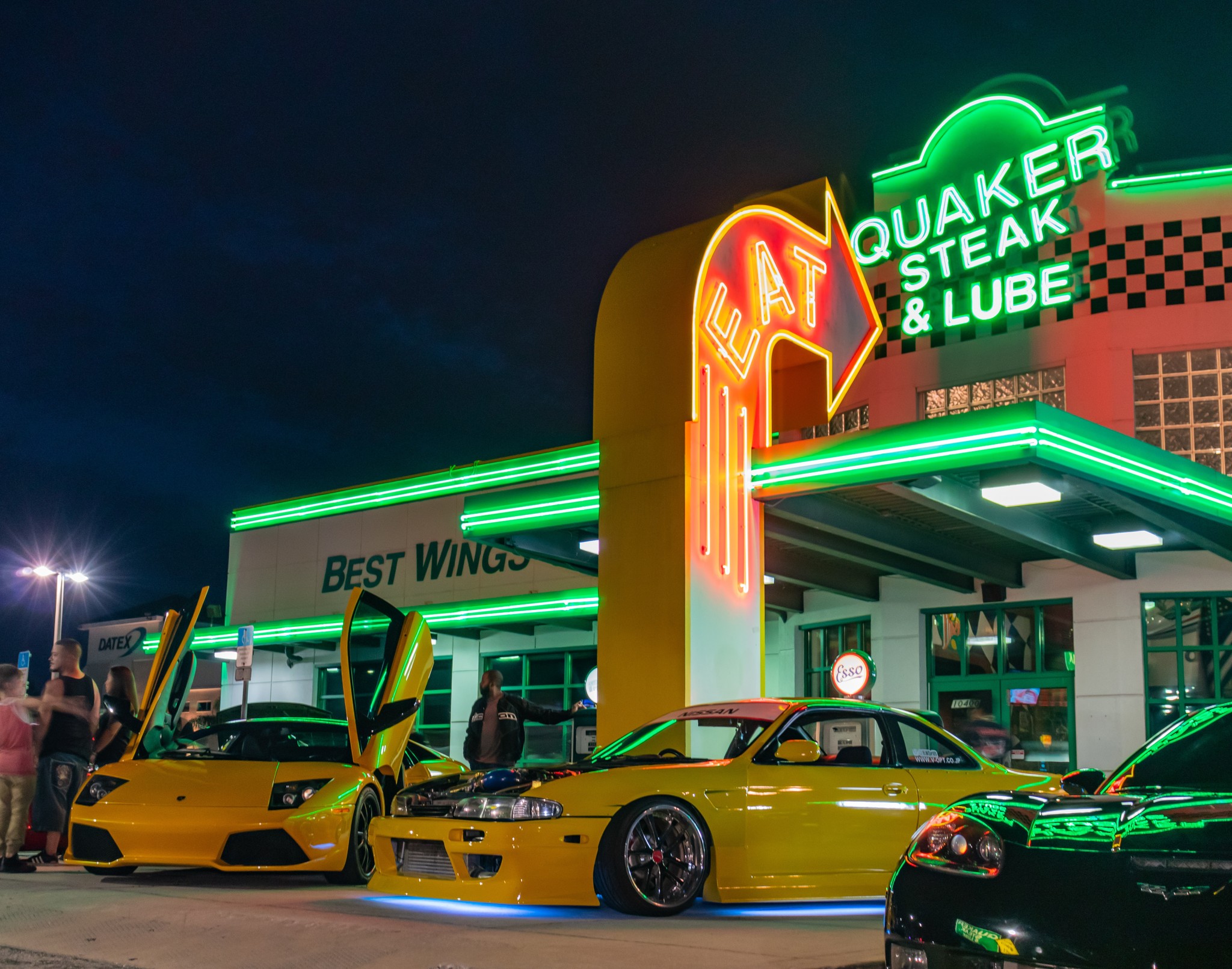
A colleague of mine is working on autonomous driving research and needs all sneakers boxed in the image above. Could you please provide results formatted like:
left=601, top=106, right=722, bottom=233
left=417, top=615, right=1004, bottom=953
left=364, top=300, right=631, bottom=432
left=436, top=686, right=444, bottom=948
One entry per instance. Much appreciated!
left=26, top=850, right=60, bottom=866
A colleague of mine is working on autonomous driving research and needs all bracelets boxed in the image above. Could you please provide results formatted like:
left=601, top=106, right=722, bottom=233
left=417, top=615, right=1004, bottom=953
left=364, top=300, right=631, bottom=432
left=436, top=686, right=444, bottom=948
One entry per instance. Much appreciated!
left=92, top=750, right=98, bottom=756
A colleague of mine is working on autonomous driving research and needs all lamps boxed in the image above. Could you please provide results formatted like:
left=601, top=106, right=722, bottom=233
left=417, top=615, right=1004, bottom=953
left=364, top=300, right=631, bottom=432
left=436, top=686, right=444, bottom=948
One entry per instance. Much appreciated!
left=1091, top=520, right=1165, bottom=551
left=977, top=467, right=1068, bottom=508
left=213, top=648, right=238, bottom=660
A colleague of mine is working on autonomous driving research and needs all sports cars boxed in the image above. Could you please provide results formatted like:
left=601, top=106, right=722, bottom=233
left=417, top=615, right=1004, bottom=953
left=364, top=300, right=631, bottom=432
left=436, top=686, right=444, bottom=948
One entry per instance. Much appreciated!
left=884, top=704, right=1232, bottom=969
left=369, top=696, right=1089, bottom=917
left=57, top=588, right=474, bottom=875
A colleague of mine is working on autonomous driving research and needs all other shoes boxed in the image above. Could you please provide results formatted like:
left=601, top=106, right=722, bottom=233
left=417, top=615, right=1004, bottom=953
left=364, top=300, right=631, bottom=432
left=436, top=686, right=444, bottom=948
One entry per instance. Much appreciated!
left=0, top=853, right=36, bottom=872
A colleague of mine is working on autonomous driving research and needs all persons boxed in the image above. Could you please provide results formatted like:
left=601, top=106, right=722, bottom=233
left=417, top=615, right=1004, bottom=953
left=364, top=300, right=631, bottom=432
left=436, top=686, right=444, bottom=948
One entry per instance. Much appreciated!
left=90, top=665, right=138, bottom=769
left=24, top=640, right=100, bottom=867
left=462, top=670, right=587, bottom=772
left=0, top=663, right=90, bottom=873
left=961, top=697, right=1007, bottom=763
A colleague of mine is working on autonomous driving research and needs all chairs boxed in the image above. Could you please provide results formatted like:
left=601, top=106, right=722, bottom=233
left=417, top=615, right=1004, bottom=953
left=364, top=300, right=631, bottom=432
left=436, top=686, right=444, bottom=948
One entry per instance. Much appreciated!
left=835, top=744, right=875, bottom=765
